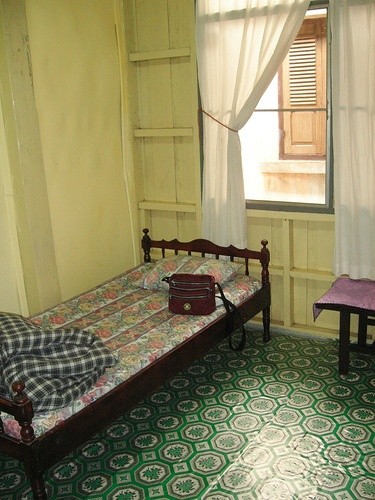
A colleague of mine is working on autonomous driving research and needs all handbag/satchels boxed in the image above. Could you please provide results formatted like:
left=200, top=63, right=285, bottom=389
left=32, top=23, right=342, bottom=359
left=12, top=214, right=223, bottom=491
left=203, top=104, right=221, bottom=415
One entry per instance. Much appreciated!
left=169, top=274, right=216, bottom=315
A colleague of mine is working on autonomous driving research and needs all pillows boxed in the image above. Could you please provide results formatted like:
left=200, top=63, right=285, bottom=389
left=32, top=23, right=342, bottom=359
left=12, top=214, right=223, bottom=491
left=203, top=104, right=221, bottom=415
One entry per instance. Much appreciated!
left=130, top=255, right=243, bottom=292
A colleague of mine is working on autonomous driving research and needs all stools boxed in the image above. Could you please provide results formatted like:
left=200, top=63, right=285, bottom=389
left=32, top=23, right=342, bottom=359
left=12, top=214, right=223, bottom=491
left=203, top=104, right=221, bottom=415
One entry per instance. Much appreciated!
left=312, top=274, right=375, bottom=375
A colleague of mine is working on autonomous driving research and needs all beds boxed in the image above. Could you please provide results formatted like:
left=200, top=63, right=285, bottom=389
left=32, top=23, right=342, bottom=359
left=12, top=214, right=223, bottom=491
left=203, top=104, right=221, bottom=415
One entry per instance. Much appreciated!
left=0, top=228, right=271, bottom=500
